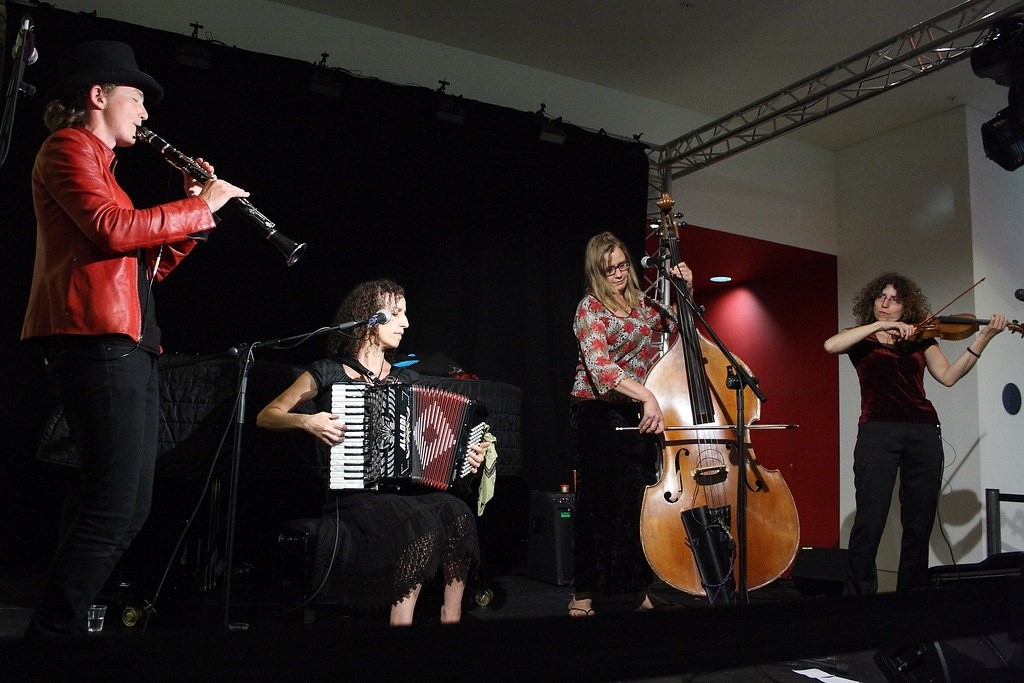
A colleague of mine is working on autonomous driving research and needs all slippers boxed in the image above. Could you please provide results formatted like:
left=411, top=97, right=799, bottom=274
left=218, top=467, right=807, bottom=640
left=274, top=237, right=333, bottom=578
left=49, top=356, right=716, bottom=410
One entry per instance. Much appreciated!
left=567, top=602, right=595, bottom=617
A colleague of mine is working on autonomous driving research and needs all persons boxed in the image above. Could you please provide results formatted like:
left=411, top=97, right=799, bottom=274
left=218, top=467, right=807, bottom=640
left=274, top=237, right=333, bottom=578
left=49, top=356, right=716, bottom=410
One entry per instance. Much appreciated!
left=568, top=232, right=693, bottom=617
left=257, top=280, right=492, bottom=626
left=825, top=272, right=1009, bottom=598
left=23, top=40, right=252, bottom=638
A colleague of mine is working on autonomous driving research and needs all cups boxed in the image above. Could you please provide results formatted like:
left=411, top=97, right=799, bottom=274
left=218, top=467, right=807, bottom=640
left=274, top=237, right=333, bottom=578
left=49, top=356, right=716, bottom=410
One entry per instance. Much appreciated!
left=88, top=605, right=107, bottom=631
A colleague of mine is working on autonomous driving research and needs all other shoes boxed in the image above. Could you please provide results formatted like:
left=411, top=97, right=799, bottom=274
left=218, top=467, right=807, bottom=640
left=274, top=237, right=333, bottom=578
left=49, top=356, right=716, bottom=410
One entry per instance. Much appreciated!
left=35, top=618, right=81, bottom=639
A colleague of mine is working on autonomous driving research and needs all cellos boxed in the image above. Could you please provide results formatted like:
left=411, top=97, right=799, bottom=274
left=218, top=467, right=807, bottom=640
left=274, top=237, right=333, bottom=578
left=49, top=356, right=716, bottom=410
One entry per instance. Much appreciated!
left=637, top=190, right=800, bottom=610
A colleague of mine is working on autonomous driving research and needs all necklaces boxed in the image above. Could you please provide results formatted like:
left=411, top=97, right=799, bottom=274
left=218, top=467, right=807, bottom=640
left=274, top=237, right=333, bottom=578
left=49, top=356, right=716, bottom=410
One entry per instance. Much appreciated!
left=378, top=358, right=385, bottom=379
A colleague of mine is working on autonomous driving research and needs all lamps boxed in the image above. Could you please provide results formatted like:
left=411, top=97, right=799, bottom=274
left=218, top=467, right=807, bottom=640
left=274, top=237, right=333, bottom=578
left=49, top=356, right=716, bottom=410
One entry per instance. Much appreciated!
left=969, top=19, right=1024, bottom=173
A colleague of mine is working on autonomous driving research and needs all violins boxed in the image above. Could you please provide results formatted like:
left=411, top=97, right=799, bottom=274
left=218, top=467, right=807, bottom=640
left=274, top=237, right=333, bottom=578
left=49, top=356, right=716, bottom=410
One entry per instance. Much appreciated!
left=892, top=313, right=1024, bottom=342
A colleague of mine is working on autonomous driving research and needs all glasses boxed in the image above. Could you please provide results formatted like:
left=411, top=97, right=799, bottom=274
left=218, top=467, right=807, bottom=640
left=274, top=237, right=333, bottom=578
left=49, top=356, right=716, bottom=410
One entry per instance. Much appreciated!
left=876, top=293, right=901, bottom=304
left=601, top=261, right=629, bottom=275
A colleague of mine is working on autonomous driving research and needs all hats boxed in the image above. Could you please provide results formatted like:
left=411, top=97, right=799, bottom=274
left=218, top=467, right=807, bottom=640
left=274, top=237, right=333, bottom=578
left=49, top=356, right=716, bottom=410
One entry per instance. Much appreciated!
left=45, top=40, right=163, bottom=105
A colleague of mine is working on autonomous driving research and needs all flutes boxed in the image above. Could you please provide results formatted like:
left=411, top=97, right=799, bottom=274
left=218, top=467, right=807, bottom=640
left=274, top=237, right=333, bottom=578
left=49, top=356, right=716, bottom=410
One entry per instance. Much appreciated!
left=135, top=126, right=309, bottom=267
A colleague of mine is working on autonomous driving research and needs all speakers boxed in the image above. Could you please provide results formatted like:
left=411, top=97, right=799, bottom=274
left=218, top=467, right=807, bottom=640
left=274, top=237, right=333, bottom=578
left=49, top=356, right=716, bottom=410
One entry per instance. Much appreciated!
left=512, top=489, right=577, bottom=585
left=874, top=558, right=1024, bottom=683
left=791, top=547, right=850, bottom=599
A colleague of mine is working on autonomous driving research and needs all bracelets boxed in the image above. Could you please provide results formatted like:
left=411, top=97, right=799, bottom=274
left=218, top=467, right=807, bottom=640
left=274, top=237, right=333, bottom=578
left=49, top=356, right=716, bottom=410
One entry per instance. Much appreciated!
left=967, top=346, right=980, bottom=358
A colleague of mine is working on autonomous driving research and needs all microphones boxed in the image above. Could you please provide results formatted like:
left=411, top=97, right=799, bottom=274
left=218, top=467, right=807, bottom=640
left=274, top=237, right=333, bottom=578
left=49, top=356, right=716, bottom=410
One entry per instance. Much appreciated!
left=12, top=15, right=39, bottom=66
left=365, top=309, right=391, bottom=327
left=641, top=254, right=673, bottom=268
left=1014, top=288, right=1024, bottom=302
